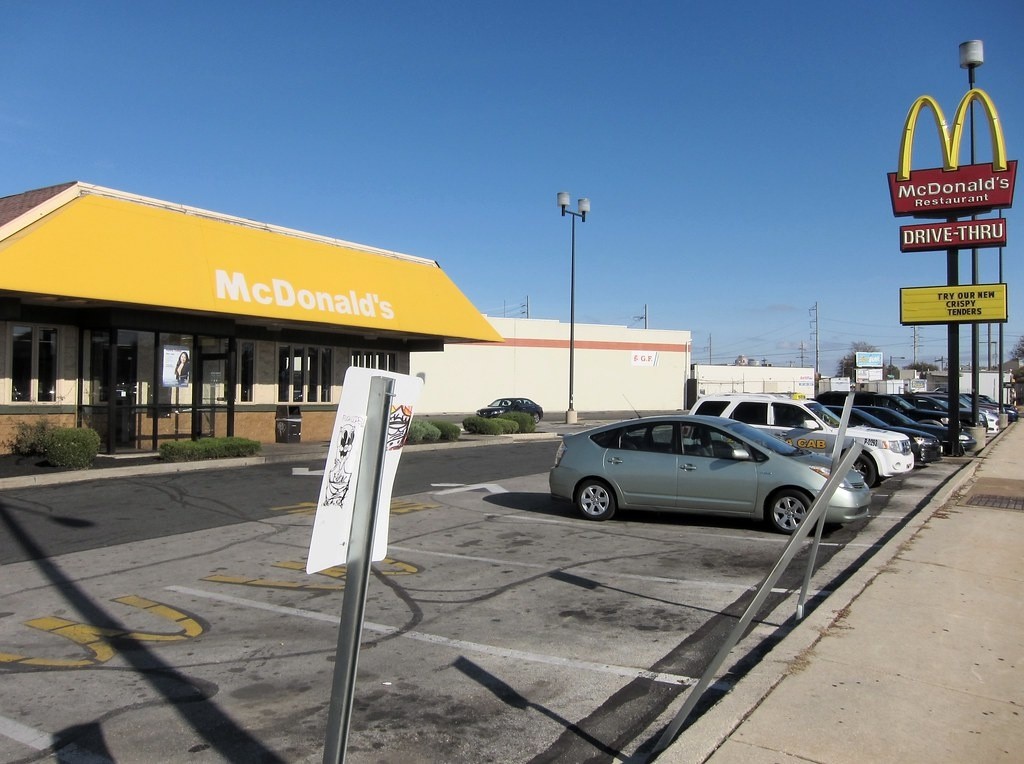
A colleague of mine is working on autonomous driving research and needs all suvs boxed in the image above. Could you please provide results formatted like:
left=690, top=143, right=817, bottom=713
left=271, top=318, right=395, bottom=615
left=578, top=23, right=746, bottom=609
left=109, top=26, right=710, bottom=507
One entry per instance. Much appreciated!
left=681, top=393, right=915, bottom=489
left=817, top=390, right=963, bottom=433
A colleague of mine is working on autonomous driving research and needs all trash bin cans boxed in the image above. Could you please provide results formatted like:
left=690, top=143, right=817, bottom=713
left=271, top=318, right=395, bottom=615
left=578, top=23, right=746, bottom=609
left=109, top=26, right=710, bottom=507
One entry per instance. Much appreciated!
left=275, top=405, right=303, bottom=443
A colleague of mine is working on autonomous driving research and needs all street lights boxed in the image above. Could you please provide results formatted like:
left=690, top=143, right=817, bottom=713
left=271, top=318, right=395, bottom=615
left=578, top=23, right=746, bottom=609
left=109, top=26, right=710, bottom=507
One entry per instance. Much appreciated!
left=890, top=355, right=905, bottom=375
left=557, top=191, right=591, bottom=425
left=959, top=39, right=987, bottom=453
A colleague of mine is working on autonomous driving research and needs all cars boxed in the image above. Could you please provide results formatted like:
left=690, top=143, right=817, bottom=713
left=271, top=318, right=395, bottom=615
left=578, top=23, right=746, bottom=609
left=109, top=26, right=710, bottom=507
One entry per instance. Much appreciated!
left=892, top=386, right=1019, bottom=428
left=849, top=405, right=977, bottom=453
left=809, top=405, right=944, bottom=463
left=549, top=415, right=871, bottom=535
left=476, top=398, right=544, bottom=424
